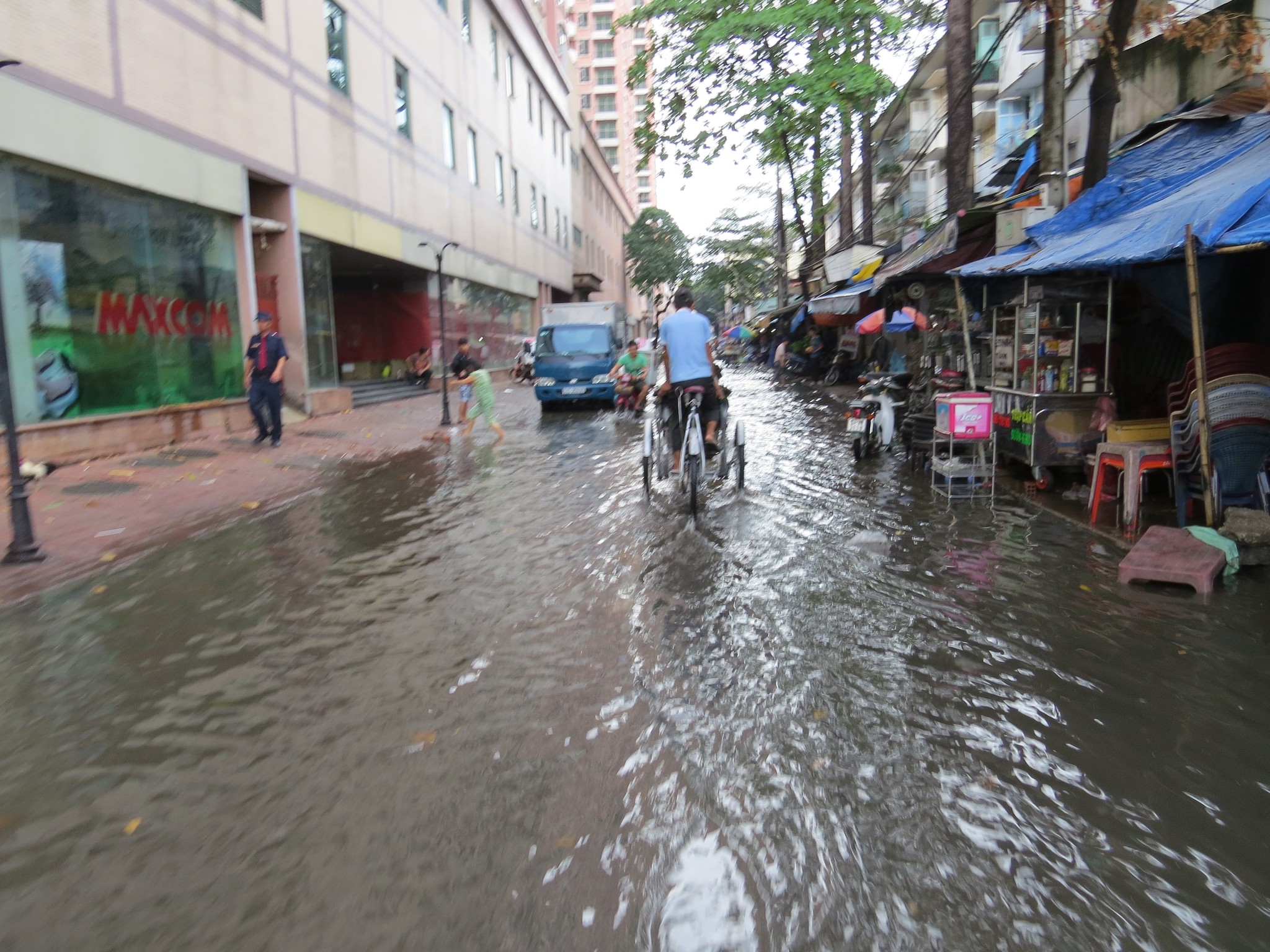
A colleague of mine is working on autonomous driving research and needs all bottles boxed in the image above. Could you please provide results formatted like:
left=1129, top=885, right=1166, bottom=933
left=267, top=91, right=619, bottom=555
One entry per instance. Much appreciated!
left=1020, top=358, right=1074, bottom=393
left=1078, top=366, right=1097, bottom=393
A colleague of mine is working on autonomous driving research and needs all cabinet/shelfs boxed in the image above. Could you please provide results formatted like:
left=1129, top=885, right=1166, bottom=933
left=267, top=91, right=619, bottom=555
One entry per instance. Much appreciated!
left=932, top=427, right=996, bottom=509
left=991, top=300, right=1080, bottom=394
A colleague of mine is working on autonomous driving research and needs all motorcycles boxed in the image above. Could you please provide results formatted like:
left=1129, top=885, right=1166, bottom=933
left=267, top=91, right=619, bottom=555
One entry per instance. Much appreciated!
left=845, top=370, right=914, bottom=463
left=607, top=369, right=648, bottom=419
left=781, top=352, right=867, bottom=388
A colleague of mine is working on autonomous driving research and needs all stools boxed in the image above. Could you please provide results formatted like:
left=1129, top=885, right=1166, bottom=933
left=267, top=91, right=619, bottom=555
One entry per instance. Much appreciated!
left=1086, top=440, right=1172, bottom=537
left=1118, top=524, right=1227, bottom=591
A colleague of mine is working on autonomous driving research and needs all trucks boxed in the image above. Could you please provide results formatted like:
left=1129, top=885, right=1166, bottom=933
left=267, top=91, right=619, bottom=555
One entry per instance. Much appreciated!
left=533, top=301, right=629, bottom=412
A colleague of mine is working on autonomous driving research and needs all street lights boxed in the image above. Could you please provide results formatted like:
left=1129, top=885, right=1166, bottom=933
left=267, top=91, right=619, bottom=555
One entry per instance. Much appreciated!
left=414, top=241, right=459, bottom=424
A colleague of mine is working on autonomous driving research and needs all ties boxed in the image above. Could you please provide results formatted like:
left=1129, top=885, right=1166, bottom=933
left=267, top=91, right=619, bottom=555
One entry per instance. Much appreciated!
left=259, top=335, right=268, bottom=371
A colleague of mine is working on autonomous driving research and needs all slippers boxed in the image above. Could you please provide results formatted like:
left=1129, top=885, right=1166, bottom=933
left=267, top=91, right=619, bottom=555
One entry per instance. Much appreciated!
left=704, top=440, right=720, bottom=453
left=671, top=463, right=680, bottom=477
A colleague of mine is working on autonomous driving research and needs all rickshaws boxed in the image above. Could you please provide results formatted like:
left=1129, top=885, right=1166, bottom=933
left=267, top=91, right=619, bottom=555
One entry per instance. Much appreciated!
left=643, top=381, right=749, bottom=518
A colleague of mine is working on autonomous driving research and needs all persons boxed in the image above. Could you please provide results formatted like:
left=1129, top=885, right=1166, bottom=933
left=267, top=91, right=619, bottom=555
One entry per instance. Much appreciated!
left=404, top=338, right=504, bottom=442
left=604, top=287, right=887, bottom=478
left=243, top=311, right=288, bottom=449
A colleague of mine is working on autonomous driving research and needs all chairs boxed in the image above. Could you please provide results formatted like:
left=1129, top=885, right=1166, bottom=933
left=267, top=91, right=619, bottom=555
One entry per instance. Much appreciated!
left=1166, top=341, right=1270, bottom=527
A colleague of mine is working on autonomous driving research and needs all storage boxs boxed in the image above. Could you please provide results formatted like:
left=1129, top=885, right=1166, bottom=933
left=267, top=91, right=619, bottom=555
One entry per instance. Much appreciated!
left=1107, top=418, right=1170, bottom=442
left=343, top=356, right=406, bottom=380
left=934, top=391, right=994, bottom=438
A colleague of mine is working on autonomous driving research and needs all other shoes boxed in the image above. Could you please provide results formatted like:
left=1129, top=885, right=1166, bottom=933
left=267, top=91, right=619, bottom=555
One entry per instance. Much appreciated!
left=254, top=433, right=267, bottom=444
left=271, top=438, right=280, bottom=447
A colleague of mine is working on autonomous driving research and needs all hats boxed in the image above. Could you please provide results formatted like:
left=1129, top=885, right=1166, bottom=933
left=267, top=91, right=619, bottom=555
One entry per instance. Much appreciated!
left=254, top=312, right=270, bottom=321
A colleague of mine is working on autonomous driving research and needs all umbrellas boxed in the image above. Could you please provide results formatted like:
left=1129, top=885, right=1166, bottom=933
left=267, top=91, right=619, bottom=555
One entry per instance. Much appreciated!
left=723, top=324, right=758, bottom=345
left=852, top=302, right=930, bottom=335
